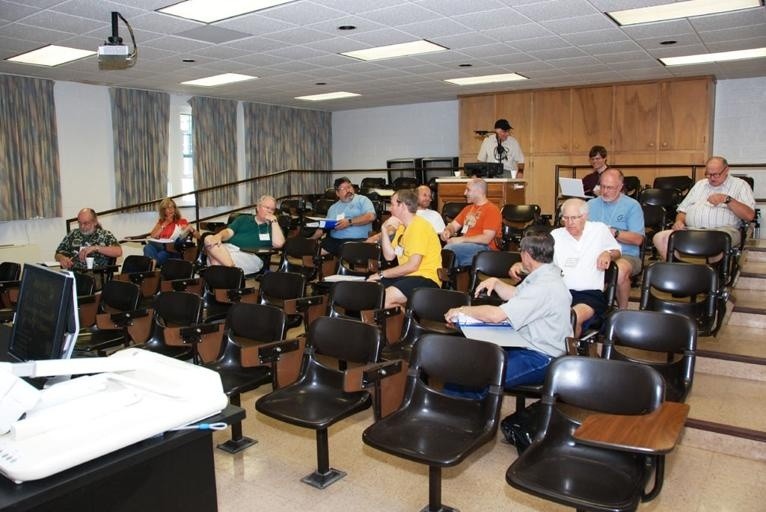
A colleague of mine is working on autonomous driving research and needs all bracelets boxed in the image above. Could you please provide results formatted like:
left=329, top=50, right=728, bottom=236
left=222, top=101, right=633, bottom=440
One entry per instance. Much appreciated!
left=376, top=270, right=385, bottom=281
left=460, top=304, right=464, bottom=312
left=95, top=245, right=98, bottom=254
left=270, top=218, right=278, bottom=223
left=348, top=218, right=352, bottom=226
left=191, top=228, right=195, bottom=234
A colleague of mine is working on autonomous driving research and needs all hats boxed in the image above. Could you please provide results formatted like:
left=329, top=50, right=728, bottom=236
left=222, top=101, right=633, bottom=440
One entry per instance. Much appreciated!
left=495, top=119, right=513, bottom=131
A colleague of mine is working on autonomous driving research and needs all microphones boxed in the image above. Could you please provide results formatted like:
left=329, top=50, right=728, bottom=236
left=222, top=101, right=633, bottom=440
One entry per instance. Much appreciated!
left=474, top=129, right=508, bottom=163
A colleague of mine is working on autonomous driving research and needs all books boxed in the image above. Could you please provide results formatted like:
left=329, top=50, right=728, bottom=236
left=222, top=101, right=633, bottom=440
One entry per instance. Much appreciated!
left=303, top=216, right=338, bottom=229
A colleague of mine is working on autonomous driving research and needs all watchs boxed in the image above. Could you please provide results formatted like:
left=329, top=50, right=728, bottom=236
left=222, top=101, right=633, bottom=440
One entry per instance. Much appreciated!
left=725, top=196, right=732, bottom=205
left=615, top=229, right=619, bottom=239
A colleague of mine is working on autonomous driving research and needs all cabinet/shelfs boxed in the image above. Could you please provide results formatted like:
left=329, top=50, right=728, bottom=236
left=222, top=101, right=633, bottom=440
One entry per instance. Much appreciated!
left=457, top=73, right=717, bottom=224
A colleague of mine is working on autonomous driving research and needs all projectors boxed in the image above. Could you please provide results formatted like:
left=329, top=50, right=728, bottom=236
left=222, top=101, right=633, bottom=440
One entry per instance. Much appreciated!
left=94, top=43, right=132, bottom=71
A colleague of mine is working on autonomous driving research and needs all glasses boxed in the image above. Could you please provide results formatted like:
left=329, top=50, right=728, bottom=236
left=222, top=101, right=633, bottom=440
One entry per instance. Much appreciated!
left=562, top=216, right=581, bottom=222
left=337, top=185, right=352, bottom=191
left=705, top=166, right=727, bottom=179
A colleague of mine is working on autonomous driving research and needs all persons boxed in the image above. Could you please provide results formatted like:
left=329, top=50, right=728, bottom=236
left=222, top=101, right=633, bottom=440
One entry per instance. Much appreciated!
left=652, top=156, right=757, bottom=264
left=439, top=232, right=575, bottom=401
left=582, top=145, right=611, bottom=195
left=143, top=197, right=201, bottom=272
left=363, top=185, right=446, bottom=245
left=438, top=179, right=504, bottom=275
left=202, top=195, right=287, bottom=276
left=586, top=168, right=646, bottom=310
left=309, top=176, right=378, bottom=259
left=364, top=189, right=444, bottom=312
left=508, top=197, right=622, bottom=339
left=54, top=208, right=123, bottom=291
left=477, top=119, right=526, bottom=178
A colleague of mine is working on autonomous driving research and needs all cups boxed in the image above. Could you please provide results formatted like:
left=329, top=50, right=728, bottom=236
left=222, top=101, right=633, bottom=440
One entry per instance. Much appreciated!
left=454, top=171, right=461, bottom=178
left=511, top=171, right=517, bottom=179
left=86, top=258, right=94, bottom=269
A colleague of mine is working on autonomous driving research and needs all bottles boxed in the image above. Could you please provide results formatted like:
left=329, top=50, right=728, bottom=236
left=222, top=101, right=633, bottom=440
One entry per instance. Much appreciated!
left=753, top=223, right=760, bottom=239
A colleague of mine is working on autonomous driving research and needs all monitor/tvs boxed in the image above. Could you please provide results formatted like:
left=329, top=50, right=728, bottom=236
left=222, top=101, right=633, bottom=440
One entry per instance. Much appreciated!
left=7, top=263, right=75, bottom=385
